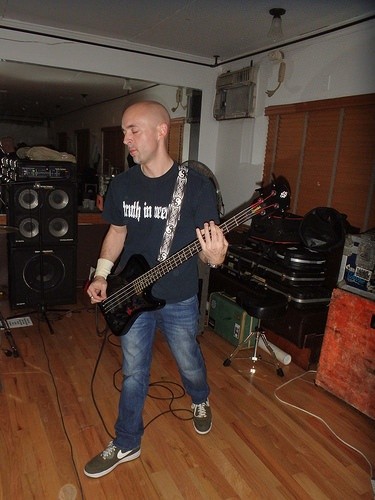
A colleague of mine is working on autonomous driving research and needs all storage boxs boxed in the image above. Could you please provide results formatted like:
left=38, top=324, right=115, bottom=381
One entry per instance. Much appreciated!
left=207, top=214, right=375, bottom=421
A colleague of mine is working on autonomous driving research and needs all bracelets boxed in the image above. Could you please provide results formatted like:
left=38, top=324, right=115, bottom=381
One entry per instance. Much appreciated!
left=94, top=258, right=114, bottom=280
left=207, top=262, right=219, bottom=269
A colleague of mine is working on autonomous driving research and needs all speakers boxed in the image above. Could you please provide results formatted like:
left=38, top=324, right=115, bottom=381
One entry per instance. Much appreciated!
left=4, top=179, right=78, bottom=246
left=9, top=246, right=77, bottom=308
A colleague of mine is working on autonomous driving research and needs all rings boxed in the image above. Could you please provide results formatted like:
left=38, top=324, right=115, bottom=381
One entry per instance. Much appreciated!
left=90, top=296, right=93, bottom=298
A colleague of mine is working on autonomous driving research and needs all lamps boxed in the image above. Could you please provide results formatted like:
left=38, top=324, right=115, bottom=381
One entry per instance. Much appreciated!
left=81, top=94, right=88, bottom=103
left=268, top=8, right=286, bottom=40
left=124, top=79, right=132, bottom=90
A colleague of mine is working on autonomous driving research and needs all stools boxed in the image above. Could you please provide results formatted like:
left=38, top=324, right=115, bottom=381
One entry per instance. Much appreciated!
left=223, top=290, right=285, bottom=376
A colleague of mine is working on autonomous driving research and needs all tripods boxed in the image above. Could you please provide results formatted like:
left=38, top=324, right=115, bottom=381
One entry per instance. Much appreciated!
left=5, top=188, right=69, bottom=334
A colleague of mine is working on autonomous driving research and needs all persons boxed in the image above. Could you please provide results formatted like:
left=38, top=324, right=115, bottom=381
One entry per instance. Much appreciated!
left=0, top=136, right=19, bottom=159
left=82, top=102, right=229, bottom=479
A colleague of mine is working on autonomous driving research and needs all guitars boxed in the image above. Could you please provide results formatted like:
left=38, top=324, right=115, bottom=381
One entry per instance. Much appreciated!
left=97, top=178, right=291, bottom=337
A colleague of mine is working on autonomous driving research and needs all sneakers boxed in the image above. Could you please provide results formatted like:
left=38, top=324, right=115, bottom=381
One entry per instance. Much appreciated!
left=191, top=398, right=212, bottom=434
left=83, top=441, right=141, bottom=478
